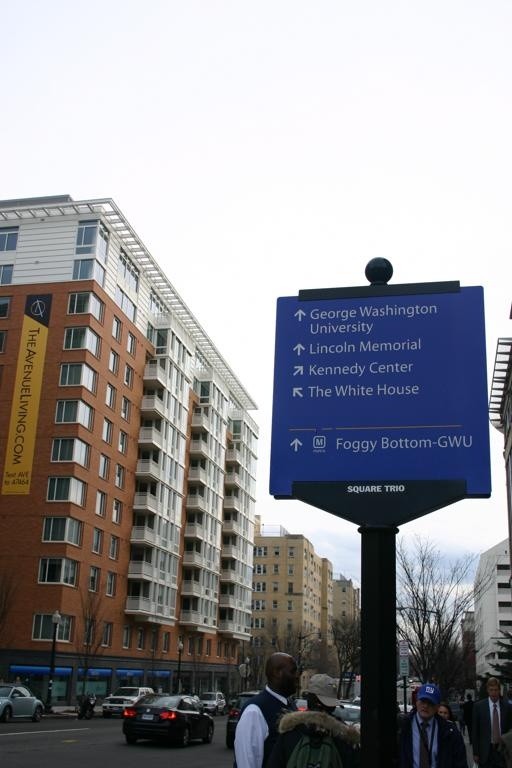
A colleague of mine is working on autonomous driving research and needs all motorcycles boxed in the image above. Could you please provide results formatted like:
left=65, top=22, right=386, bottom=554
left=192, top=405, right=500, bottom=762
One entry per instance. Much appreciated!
left=76, top=691, right=97, bottom=718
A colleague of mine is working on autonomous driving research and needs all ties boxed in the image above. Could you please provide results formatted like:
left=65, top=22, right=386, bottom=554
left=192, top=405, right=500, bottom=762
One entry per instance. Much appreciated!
left=420, top=720, right=431, bottom=767
left=493, top=702, right=501, bottom=745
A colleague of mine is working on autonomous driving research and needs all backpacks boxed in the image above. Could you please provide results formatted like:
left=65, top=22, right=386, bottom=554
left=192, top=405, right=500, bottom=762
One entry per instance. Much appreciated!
left=285, top=727, right=346, bottom=768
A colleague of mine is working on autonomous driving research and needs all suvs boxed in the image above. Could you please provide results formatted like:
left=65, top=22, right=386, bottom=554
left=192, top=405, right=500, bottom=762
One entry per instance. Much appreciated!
left=199, top=691, right=226, bottom=715
left=226, top=689, right=264, bottom=748
left=102, top=685, right=155, bottom=718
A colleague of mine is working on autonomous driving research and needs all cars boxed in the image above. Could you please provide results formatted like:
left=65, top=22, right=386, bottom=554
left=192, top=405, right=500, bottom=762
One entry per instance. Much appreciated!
left=294, top=693, right=414, bottom=730
left=121, top=692, right=215, bottom=745
left=398, top=676, right=422, bottom=687
left=0, top=681, right=44, bottom=721
left=226, top=694, right=239, bottom=711
left=445, top=701, right=464, bottom=721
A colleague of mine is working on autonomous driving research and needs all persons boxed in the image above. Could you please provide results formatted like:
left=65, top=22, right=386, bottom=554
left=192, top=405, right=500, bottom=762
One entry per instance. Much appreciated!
left=232, top=651, right=299, bottom=768
left=265, top=673, right=361, bottom=768
left=397, top=674, right=512, bottom=768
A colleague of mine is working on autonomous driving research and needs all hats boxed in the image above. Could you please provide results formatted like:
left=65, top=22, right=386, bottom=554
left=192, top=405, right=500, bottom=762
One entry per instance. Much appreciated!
left=304, top=673, right=345, bottom=709
left=418, top=684, right=441, bottom=705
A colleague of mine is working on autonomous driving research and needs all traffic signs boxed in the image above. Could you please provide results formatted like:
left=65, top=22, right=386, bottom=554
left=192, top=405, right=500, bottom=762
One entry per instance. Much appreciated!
left=269, top=284, right=492, bottom=498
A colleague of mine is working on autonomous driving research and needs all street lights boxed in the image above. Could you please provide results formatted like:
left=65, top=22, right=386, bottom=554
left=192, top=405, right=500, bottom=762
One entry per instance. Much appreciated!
left=245, top=656, right=250, bottom=690
left=298, top=630, right=322, bottom=696
left=43, top=608, right=61, bottom=714
left=176, top=640, right=185, bottom=694
left=397, top=605, right=442, bottom=650
